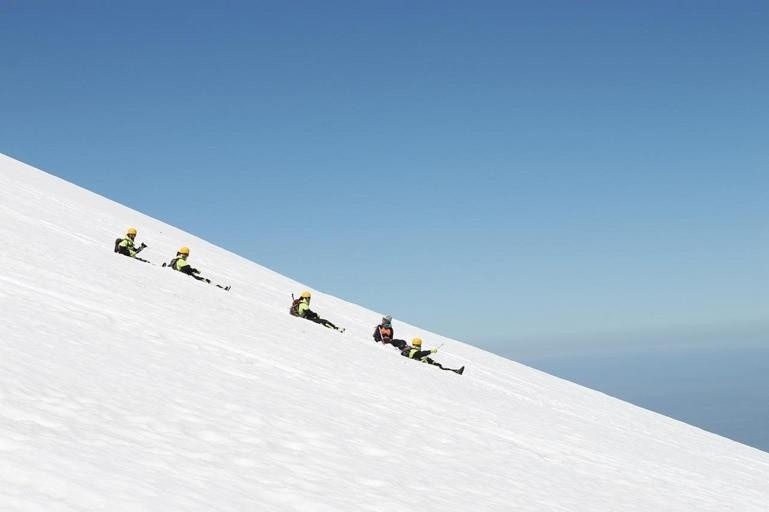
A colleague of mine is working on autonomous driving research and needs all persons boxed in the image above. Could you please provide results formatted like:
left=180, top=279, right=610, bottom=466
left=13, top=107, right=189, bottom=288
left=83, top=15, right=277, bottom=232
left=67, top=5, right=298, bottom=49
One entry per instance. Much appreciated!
left=118, top=227, right=167, bottom=268
left=173, top=246, right=231, bottom=290
left=408, top=337, right=464, bottom=376
left=373, top=315, right=411, bottom=356
left=297, top=292, right=345, bottom=334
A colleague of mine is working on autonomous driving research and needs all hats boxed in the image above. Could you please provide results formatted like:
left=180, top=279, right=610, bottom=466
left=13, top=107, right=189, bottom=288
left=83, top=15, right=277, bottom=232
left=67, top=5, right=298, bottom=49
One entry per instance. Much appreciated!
left=383, top=315, right=392, bottom=322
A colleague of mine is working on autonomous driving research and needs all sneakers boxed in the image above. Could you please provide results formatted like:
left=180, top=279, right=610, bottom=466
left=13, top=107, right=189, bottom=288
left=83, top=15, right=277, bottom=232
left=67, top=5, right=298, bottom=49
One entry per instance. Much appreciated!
left=224, top=287, right=228, bottom=290
left=458, top=366, right=464, bottom=374
left=162, top=263, right=166, bottom=267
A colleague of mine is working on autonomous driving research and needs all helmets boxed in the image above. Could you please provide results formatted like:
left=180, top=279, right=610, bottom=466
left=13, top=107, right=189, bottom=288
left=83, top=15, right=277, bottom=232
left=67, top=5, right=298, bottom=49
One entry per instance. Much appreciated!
left=302, top=291, right=310, bottom=298
left=412, top=338, right=421, bottom=346
left=178, top=247, right=190, bottom=254
left=127, top=228, right=137, bottom=235
